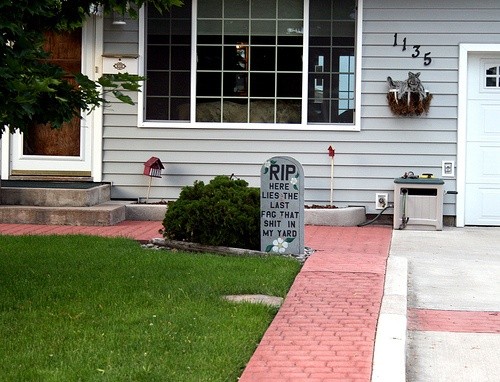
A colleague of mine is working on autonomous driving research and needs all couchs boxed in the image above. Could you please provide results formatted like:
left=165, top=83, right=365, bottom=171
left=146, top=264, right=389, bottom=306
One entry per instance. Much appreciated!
left=178, top=100, right=302, bottom=124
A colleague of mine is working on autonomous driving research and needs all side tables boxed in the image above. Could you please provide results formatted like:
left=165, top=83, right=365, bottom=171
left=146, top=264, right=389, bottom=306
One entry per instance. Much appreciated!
left=394, top=176, right=446, bottom=231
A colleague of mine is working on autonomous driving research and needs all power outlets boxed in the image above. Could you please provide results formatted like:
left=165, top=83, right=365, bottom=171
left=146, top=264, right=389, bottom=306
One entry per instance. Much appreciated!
left=376, top=192, right=388, bottom=209
